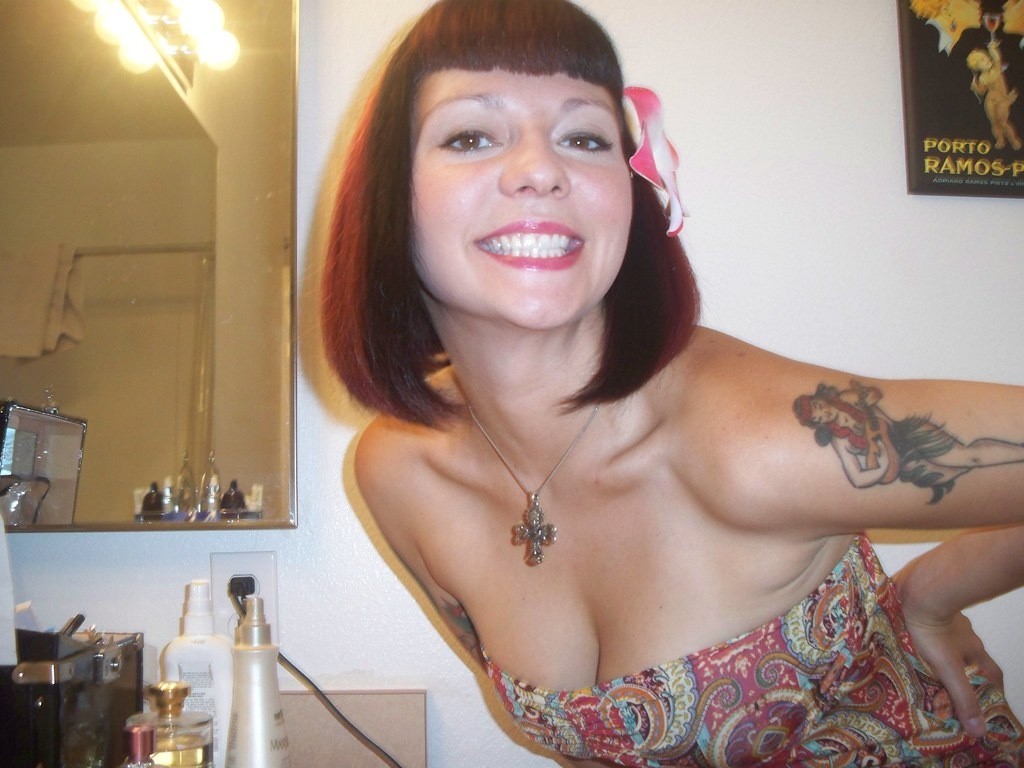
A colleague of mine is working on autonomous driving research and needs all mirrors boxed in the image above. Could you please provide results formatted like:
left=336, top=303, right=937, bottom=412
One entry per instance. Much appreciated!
left=0, top=0, right=299, bottom=531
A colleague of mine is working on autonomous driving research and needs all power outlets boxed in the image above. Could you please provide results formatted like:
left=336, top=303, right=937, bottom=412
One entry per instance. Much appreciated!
left=209, top=552, right=279, bottom=655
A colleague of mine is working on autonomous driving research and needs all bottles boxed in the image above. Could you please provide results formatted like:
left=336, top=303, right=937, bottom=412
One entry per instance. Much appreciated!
left=142, top=449, right=246, bottom=523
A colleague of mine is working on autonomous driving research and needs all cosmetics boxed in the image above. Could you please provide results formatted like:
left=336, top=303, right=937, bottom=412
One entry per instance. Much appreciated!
left=120, top=579, right=290, bottom=768
left=134, top=452, right=259, bottom=522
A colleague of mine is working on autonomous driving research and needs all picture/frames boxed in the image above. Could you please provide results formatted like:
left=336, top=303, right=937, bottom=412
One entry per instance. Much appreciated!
left=896, top=0, right=1024, bottom=197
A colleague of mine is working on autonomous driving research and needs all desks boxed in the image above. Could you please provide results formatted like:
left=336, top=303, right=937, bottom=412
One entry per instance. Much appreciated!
left=275, top=689, right=426, bottom=768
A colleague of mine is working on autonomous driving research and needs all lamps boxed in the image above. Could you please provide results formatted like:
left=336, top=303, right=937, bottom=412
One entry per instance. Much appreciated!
left=77, top=1, right=241, bottom=76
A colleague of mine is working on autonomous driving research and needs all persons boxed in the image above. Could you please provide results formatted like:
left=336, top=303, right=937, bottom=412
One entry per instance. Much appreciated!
left=316, top=0, right=1024, bottom=768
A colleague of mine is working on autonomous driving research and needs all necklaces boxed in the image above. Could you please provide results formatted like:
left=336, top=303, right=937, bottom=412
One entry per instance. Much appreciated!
left=465, top=404, right=599, bottom=567
